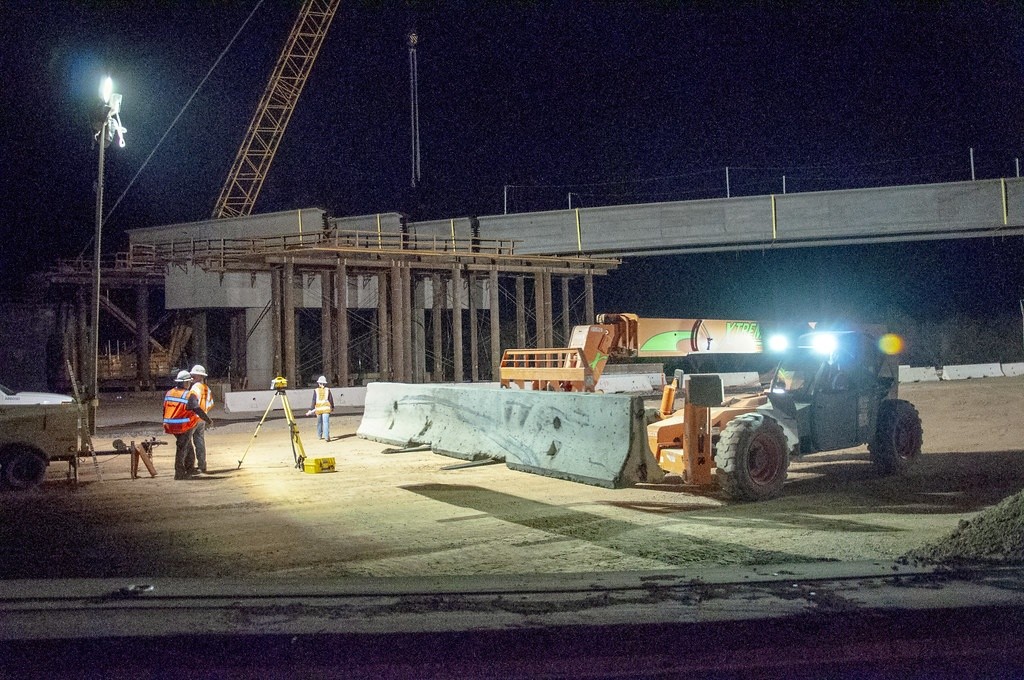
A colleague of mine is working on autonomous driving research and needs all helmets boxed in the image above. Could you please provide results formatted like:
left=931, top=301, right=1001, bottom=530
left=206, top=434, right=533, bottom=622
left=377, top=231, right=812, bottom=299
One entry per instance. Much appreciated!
left=175, top=370, right=193, bottom=382
left=317, top=376, right=327, bottom=384
left=190, top=365, right=208, bottom=376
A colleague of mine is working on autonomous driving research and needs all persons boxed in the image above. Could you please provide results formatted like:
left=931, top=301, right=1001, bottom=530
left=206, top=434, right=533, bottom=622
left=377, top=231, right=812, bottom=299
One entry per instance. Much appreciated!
left=309, top=376, right=334, bottom=442
left=162, top=365, right=215, bottom=480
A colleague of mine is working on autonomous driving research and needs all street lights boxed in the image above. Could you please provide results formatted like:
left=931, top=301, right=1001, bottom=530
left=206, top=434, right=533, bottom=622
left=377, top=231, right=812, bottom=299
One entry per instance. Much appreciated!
left=87, top=73, right=115, bottom=438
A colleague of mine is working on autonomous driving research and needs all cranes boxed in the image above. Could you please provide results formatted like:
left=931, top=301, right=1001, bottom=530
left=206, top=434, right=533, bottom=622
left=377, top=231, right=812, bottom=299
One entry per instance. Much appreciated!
left=98, top=2, right=340, bottom=379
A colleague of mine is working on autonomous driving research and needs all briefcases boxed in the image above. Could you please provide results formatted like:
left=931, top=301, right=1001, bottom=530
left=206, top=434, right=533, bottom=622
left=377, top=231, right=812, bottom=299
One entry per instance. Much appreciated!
left=303, top=456, right=336, bottom=474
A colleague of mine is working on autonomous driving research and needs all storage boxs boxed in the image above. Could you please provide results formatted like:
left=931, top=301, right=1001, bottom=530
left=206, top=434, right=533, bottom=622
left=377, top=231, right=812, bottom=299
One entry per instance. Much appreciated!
left=0, top=392, right=79, bottom=456
left=303, top=456, right=337, bottom=473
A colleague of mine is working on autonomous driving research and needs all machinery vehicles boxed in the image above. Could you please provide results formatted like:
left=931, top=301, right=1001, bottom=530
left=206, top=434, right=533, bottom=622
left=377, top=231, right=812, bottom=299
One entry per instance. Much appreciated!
left=497, top=309, right=925, bottom=503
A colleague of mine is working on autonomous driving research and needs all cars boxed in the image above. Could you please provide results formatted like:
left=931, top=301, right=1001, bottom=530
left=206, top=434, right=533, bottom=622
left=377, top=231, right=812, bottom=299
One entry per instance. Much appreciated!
left=0, top=385, right=84, bottom=490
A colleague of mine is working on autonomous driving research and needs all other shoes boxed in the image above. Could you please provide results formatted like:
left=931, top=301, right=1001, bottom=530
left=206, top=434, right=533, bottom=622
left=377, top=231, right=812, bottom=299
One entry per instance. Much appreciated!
left=174, top=468, right=199, bottom=479
left=325, top=437, right=330, bottom=441
left=317, top=435, right=322, bottom=440
